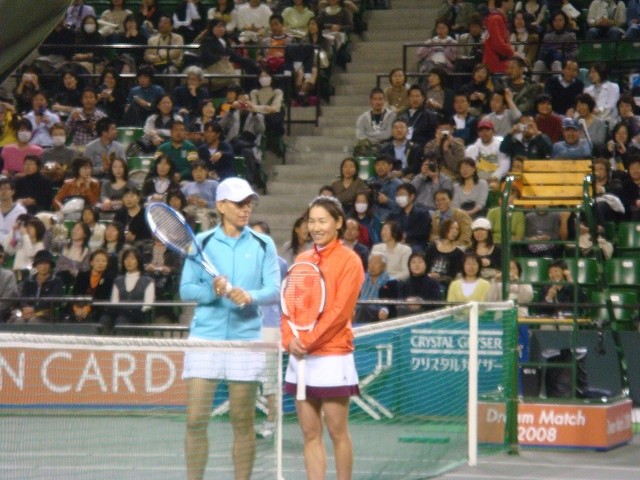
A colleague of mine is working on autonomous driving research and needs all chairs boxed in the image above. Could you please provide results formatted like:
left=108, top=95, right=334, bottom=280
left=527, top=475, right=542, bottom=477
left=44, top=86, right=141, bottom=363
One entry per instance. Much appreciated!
left=517, top=257, right=551, bottom=303
left=606, top=221, right=640, bottom=330
left=352, top=155, right=377, bottom=181
left=502, top=159, right=593, bottom=404
left=583, top=292, right=611, bottom=328
left=560, top=258, right=601, bottom=284
left=578, top=42, right=640, bottom=76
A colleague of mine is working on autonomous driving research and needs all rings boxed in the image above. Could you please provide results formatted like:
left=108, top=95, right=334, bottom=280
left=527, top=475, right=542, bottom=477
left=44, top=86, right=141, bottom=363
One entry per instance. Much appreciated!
left=216, top=287, right=220, bottom=294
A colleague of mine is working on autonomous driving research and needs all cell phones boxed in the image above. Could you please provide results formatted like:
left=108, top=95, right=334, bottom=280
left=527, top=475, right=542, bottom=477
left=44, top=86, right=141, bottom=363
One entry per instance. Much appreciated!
left=37, top=105, right=44, bottom=116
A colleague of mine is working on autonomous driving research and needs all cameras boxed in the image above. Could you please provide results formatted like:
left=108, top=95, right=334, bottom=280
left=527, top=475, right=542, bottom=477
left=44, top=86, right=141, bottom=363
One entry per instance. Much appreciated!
left=44, top=161, right=55, bottom=170
left=518, top=124, right=527, bottom=133
left=441, top=130, right=450, bottom=137
left=427, top=160, right=436, bottom=172
left=612, top=142, right=621, bottom=151
left=75, top=111, right=88, bottom=123
left=241, top=101, right=248, bottom=110
left=26, top=74, right=33, bottom=82
left=18, top=220, right=25, bottom=229
left=364, top=180, right=381, bottom=191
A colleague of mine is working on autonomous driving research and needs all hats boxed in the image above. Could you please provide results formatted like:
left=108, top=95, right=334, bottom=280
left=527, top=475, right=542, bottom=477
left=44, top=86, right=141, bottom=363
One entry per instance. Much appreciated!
left=470, top=217, right=493, bottom=231
left=562, top=117, right=582, bottom=131
left=33, top=249, right=55, bottom=267
left=478, top=120, right=494, bottom=129
left=215, top=177, right=260, bottom=204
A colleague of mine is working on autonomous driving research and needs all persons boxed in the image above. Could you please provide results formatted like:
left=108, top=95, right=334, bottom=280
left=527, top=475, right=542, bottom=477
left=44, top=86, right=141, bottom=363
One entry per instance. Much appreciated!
left=346, top=192, right=381, bottom=242
left=509, top=9, right=539, bottom=72
left=219, top=84, right=245, bottom=119
left=595, top=122, right=640, bottom=171
left=124, top=64, right=166, bottom=127
left=417, top=17, right=457, bottom=86
left=65, top=0, right=98, bottom=30
left=381, top=119, right=422, bottom=184
left=465, top=120, right=511, bottom=207
left=99, top=158, right=140, bottom=211
left=12, top=155, right=49, bottom=216
left=456, top=12, right=484, bottom=60
left=51, top=70, right=82, bottom=121
left=446, top=253, right=492, bottom=302
left=96, top=222, right=132, bottom=272
left=64, top=86, right=108, bottom=145
left=580, top=207, right=615, bottom=258
left=531, top=94, right=563, bottom=143
left=397, top=85, right=436, bottom=143
left=423, top=68, right=454, bottom=114
left=281, top=0, right=315, bottom=42
left=382, top=68, right=411, bottom=109
left=2, top=214, right=33, bottom=269
left=167, top=188, right=195, bottom=232
left=319, top=186, right=334, bottom=199
left=0, top=179, right=27, bottom=246
left=494, top=56, right=545, bottom=116
left=316, top=0, right=353, bottom=67
left=82, top=206, right=106, bottom=254
left=514, top=0, right=547, bottom=35
left=280, top=196, right=364, bottom=480
left=377, top=251, right=443, bottom=320
left=252, top=222, right=289, bottom=438
left=625, top=0, right=640, bottom=41
left=545, top=58, right=585, bottom=118
left=104, top=14, right=148, bottom=74
left=331, top=158, right=369, bottom=216
left=428, top=189, right=473, bottom=255
left=114, top=187, right=151, bottom=245
left=531, top=10, right=579, bottom=83
left=84, top=117, right=128, bottom=173
left=481, top=88, right=522, bottom=137
left=565, top=93, right=606, bottom=148
left=486, top=188, right=526, bottom=244
left=172, top=66, right=209, bottom=129
left=157, top=121, right=195, bottom=181
left=140, top=225, right=184, bottom=323
left=69, top=15, right=106, bottom=63
left=181, top=158, right=220, bottom=232
left=352, top=252, right=396, bottom=324
left=411, top=156, right=453, bottom=214
left=485, top=258, right=534, bottom=397
left=451, top=157, right=489, bottom=220
left=52, top=222, right=92, bottom=285
left=13, top=67, right=48, bottom=112
left=537, top=259, right=592, bottom=399
left=459, top=63, right=493, bottom=114
left=367, top=156, right=405, bottom=224
left=248, top=66, right=285, bottom=159
left=435, top=0, right=477, bottom=34
left=586, top=0, right=626, bottom=42
left=201, top=19, right=235, bottom=70
left=236, top=0, right=273, bottom=59
left=385, top=183, right=431, bottom=256
left=13, top=220, right=45, bottom=283
left=143, top=95, right=184, bottom=152
left=550, top=0, right=579, bottom=31
left=139, top=0, right=158, bottom=33
left=198, top=121, right=235, bottom=182
left=621, top=158, right=640, bottom=208
left=295, top=16, right=330, bottom=101
left=100, top=249, right=155, bottom=335
left=424, top=220, right=466, bottom=300
left=52, top=157, right=101, bottom=211
left=143, top=155, right=178, bottom=203
left=171, top=0, right=207, bottom=44
left=179, top=177, right=281, bottom=480
left=574, top=61, right=620, bottom=131
left=317, top=0, right=359, bottom=35
left=448, top=93, right=478, bottom=146
left=185, top=100, right=217, bottom=145
left=500, top=115, right=553, bottom=160
left=282, top=217, right=314, bottom=270
left=256, top=14, right=297, bottom=74
left=370, top=221, right=412, bottom=280
left=21, top=89, right=62, bottom=148
left=477, top=0, right=495, bottom=18
left=143, top=15, right=184, bottom=74
left=552, top=117, right=592, bottom=159
left=423, top=120, right=464, bottom=182
left=15, top=250, right=66, bottom=323
left=464, top=217, right=501, bottom=281
left=1, top=118, right=44, bottom=176
left=525, top=204, right=564, bottom=258
left=1, top=88, right=22, bottom=147
left=0, top=245, right=18, bottom=323
left=339, top=218, right=369, bottom=272
left=207, top=0, right=238, bottom=45
left=356, top=88, right=398, bottom=152
left=592, top=158, right=624, bottom=221
left=617, top=95, right=640, bottom=145
left=500, top=155, right=535, bottom=208
left=219, top=93, right=266, bottom=196
left=93, top=66, right=126, bottom=126
left=63, top=249, right=113, bottom=324
left=101, top=0, right=132, bottom=34
left=40, top=124, right=84, bottom=188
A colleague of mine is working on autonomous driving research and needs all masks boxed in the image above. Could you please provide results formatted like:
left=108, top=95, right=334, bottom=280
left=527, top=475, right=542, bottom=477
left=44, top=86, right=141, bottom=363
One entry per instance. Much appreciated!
left=17, top=131, right=32, bottom=142
left=395, top=196, right=410, bottom=207
left=259, top=77, right=271, bottom=87
left=354, top=203, right=368, bottom=214
left=52, top=136, right=66, bottom=147
left=84, top=24, right=96, bottom=34
